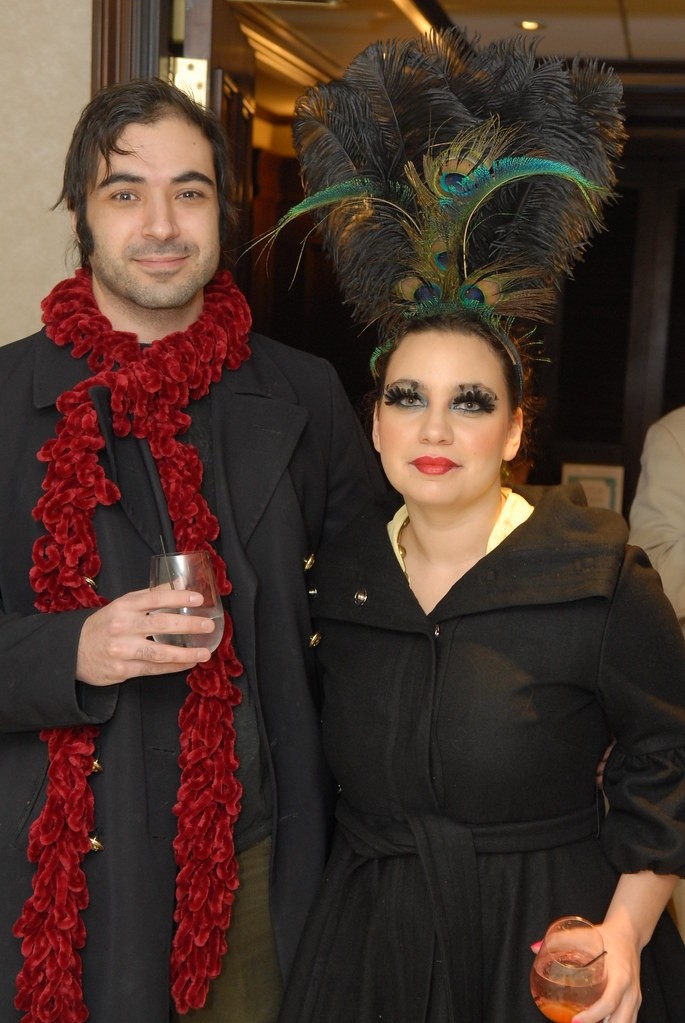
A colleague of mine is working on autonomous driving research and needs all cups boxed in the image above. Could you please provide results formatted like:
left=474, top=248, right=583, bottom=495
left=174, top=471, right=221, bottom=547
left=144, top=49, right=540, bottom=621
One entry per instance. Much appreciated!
left=148, top=550, right=225, bottom=654
left=530, top=916, right=607, bottom=1023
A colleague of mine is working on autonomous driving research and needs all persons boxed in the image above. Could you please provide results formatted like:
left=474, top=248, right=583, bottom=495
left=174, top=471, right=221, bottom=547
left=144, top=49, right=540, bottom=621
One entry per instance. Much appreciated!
left=0, top=78, right=406, bottom=1023
left=626, top=406, right=685, bottom=636
left=277, top=295, right=685, bottom=1023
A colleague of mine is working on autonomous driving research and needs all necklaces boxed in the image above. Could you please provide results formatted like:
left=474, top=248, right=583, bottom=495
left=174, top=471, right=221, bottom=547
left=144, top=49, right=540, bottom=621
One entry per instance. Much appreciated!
left=396, top=515, right=410, bottom=581
